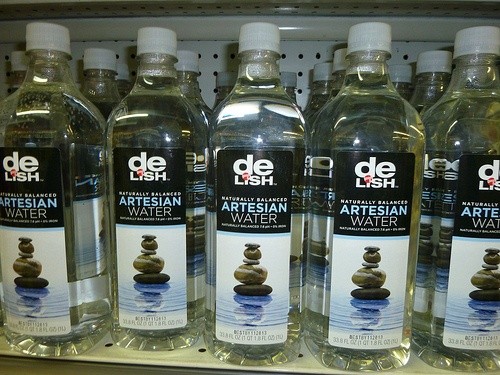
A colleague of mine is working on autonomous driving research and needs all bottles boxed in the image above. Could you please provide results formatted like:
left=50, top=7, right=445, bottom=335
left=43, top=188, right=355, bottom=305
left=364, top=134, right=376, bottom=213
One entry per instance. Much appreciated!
left=0, top=20, right=500, bottom=372
